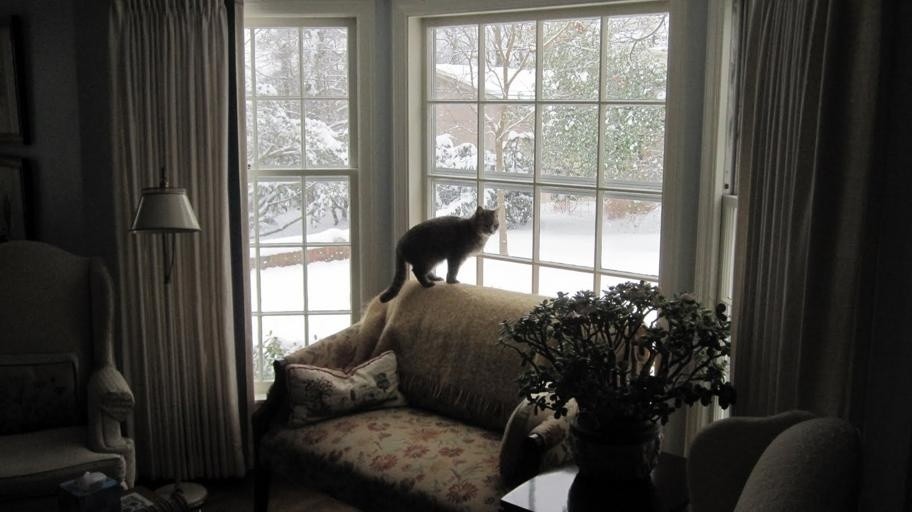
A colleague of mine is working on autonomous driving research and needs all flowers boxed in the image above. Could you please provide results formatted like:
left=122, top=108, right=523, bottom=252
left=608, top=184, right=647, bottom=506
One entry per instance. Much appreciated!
left=498, top=280, right=736, bottom=432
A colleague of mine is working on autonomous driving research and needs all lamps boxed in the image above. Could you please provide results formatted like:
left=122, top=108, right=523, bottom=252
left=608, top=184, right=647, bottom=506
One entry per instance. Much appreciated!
left=128, top=169, right=209, bottom=512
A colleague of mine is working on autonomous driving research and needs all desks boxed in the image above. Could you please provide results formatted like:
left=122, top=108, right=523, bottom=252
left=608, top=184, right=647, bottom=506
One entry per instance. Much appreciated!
left=502, top=453, right=690, bottom=512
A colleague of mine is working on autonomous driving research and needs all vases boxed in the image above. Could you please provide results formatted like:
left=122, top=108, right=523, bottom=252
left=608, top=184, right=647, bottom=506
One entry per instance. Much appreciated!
left=560, top=412, right=664, bottom=512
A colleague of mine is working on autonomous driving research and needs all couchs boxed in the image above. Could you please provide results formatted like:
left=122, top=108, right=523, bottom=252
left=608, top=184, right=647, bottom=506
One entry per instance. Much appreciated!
left=251, top=280, right=649, bottom=512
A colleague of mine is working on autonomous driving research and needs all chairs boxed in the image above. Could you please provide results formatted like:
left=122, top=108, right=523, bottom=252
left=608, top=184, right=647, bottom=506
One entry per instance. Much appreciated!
left=0, top=239, right=136, bottom=512
left=690, top=408, right=863, bottom=512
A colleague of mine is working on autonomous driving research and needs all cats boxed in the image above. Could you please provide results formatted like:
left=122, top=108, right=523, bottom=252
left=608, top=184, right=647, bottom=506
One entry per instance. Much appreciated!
left=379, top=204, right=501, bottom=304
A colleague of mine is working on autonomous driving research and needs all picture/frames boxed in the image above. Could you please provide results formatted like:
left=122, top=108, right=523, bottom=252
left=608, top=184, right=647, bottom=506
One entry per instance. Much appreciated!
left=0, top=16, right=31, bottom=147
left=0, top=155, right=36, bottom=243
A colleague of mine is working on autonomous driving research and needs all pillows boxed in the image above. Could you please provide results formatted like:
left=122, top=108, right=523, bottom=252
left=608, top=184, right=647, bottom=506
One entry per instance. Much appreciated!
left=283, top=351, right=407, bottom=428
left=0, top=353, right=80, bottom=434
left=500, top=381, right=556, bottom=471
